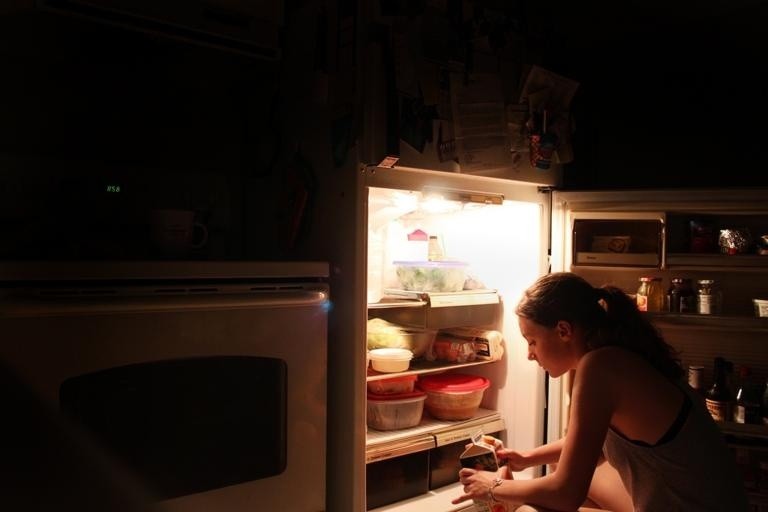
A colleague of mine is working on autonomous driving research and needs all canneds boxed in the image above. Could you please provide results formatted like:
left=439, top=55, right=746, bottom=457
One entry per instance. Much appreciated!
left=688, top=364, right=705, bottom=393
left=752, top=297, right=768, bottom=319
left=635, top=276, right=666, bottom=313
left=694, top=279, right=723, bottom=314
left=667, top=277, right=694, bottom=313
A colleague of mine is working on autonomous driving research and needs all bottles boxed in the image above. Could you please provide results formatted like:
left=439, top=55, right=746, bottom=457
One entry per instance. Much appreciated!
left=427, top=235, right=443, bottom=263
left=700, top=357, right=762, bottom=423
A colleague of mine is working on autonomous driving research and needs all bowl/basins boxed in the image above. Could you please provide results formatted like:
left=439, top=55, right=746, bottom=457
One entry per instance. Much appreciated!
left=751, top=298, right=767, bottom=317
left=589, top=235, right=632, bottom=254
left=366, top=327, right=437, bottom=362
left=368, top=350, right=414, bottom=373
left=422, top=375, right=490, bottom=421
left=390, top=261, right=470, bottom=294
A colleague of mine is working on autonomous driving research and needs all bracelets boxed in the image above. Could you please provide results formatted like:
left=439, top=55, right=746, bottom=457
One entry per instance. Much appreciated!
left=488, top=477, right=505, bottom=502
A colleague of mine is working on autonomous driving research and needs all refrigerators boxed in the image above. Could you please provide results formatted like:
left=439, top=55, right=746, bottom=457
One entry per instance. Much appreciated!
left=330, top=157, right=768, bottom=510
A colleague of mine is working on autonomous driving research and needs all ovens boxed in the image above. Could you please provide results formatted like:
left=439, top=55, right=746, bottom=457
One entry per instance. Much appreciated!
left=0, top=257, right=330, bottom=512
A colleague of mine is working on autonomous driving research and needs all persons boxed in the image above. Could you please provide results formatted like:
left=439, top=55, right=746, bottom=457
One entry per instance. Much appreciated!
left=448, top=268, right=741, bottom=510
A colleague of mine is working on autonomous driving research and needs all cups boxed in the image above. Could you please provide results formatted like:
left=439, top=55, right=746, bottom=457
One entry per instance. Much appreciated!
left=151, top=207, right=208, bottom=262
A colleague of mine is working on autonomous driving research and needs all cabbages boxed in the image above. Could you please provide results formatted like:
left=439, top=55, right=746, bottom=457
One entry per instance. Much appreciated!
left=367, top=317, right=411, bottom=353
